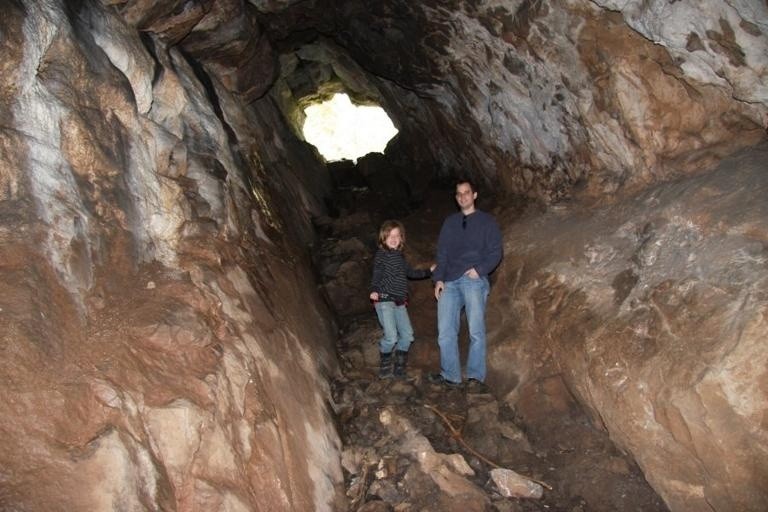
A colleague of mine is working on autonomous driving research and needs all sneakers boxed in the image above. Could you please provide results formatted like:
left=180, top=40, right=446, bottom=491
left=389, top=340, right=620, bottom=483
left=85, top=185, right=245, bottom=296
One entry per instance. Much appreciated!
left=462, top=378, right=481, bottom=393
left=427, top=373, right=457, bottom=386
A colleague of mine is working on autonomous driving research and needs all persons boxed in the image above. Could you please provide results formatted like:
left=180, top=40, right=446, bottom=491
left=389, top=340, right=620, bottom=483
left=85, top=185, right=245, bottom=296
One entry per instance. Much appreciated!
left=369, top=219, right=436, bottom=379
left=428, top=179, right=503, bottom=392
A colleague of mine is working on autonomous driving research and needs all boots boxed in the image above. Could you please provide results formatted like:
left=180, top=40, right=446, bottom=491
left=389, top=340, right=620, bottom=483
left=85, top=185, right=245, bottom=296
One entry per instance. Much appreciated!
left=392, top=350, right=416, bottom=385
left=378, top=351, right=394, bottom=379
